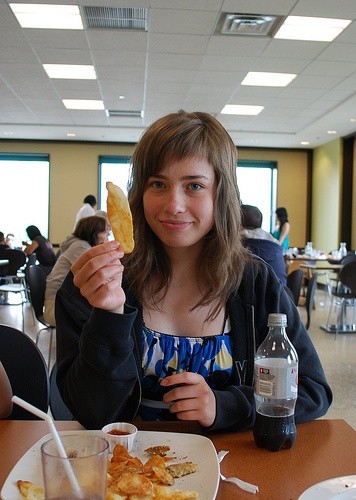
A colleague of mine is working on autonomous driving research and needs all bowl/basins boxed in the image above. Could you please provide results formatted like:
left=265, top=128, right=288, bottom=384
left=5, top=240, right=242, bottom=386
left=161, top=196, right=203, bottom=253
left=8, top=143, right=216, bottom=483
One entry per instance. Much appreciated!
left=101, top=422, right=137, bottom=454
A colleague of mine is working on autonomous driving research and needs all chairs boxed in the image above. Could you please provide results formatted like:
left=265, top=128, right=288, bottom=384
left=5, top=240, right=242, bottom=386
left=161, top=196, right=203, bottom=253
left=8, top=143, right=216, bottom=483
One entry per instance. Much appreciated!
left=0, top=248, right=59, bottom=419
left=287, top=270, right=319, bottom=331
left=325, top=259, right=356, bottom=337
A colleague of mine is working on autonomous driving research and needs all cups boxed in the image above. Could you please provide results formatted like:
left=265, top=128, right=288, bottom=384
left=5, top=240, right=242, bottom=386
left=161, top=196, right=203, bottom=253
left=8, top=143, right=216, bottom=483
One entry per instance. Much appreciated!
left=331, top=249, right=338, bottom=259
left=40, top=434, right=108, bottom=500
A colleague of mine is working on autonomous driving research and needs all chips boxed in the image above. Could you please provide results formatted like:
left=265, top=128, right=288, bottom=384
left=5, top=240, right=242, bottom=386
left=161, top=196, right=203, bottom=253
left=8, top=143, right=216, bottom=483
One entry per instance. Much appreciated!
left=17, top=444, right=198, bottom=500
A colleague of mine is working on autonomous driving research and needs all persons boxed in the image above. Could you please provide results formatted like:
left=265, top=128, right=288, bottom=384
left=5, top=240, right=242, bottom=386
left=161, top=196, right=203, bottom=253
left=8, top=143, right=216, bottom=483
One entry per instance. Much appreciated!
left=73, top=195, right=97, bottom=232
left=23, top=225, right=56, bottom=276
left=59, top=209, right=111, bottom=254
left=0, top=231, right=11, bottom=305
left=270, top=208, right=290, bottom=255
left=44, top=216, right=111, bottom=326
left=6, top=234, right=16, bottom=251
left=55, top=110, right=333, bottom=436
left=239, top=205, right=287, bottom=288
left=0, top=361, right=13, bottom=418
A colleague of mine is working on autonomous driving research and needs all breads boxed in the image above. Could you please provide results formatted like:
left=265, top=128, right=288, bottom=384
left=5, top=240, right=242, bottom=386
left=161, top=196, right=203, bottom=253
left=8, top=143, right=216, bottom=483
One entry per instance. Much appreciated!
left=106, top=182, right=134, bottom=253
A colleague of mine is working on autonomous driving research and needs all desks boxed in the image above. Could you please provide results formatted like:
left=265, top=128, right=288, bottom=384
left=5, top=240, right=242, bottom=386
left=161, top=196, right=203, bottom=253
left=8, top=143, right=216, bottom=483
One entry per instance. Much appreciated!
left=0, top=418, right=356, bottom=500
left=299, top=264, right=356, bottom=333
left=287, top=257, right=335, bottom=307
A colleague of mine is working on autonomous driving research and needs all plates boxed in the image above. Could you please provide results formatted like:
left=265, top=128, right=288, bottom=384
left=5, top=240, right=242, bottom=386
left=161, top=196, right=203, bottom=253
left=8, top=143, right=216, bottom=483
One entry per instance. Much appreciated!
left=0, top=429, right=220, bottom=500
left=297, top=474, right=356, bottom=500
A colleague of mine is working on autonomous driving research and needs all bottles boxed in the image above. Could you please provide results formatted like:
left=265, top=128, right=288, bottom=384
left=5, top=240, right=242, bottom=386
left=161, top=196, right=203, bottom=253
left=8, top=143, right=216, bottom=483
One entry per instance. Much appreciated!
left=305, top=242, right=313, bottom=257
left=338, top=242, right=347, bottom=260
left=14, top=241, right=20, bottom=249
left=252, top=313, right=298, bottom=451
